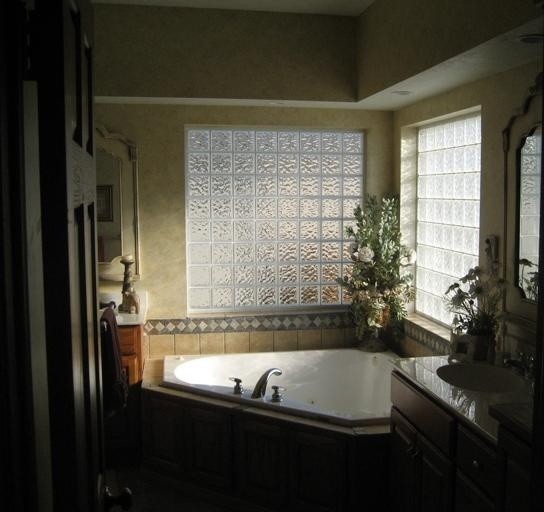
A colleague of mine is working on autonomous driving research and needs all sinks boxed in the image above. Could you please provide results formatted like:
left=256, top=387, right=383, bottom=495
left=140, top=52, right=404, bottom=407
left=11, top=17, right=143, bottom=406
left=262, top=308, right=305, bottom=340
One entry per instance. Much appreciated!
left=436, top=364, right=526, bottom=393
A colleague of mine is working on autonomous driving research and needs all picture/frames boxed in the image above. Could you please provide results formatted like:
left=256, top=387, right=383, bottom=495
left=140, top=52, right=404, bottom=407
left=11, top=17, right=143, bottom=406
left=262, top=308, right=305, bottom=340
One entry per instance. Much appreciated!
left=95, top=184, right=113, bottom=223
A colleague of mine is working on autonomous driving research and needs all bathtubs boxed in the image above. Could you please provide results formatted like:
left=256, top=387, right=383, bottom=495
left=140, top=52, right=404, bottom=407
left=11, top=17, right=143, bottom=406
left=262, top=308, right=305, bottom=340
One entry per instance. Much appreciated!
left=163, top=348, right=402, bottom=427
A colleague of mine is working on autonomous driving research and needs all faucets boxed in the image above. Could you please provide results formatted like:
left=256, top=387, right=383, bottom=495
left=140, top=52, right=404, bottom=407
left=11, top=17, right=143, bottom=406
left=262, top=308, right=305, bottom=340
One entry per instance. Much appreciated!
left=502, top=358, right=527, bottom=369
left=251, top=368, right=282, bottom=398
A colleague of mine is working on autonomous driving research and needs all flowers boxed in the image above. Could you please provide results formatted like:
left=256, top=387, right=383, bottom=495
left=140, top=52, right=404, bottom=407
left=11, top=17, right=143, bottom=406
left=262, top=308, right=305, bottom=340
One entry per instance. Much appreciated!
left=335, top=187, right=416, bottom=339
left=443, top=259, right=508, bottom=349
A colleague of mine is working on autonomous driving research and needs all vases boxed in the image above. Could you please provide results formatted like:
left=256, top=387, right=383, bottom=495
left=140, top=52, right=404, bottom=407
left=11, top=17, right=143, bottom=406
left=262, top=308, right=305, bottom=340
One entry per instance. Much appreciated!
left=354, top=320, right=388, bottom=353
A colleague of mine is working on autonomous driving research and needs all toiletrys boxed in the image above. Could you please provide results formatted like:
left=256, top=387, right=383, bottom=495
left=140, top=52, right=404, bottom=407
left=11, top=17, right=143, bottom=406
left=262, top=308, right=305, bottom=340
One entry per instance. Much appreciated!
left=494, top=321, right=511, bottom=368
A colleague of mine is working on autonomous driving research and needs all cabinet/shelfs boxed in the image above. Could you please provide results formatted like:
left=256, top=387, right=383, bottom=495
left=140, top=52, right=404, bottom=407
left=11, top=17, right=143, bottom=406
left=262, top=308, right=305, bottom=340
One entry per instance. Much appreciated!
left=384, top=367, right=543, bottom=512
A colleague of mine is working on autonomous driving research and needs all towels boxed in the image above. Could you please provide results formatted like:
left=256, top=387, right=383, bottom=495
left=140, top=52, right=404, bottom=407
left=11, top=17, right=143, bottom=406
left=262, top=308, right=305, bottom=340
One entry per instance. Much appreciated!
left=101, top=307, right=129, bottom=419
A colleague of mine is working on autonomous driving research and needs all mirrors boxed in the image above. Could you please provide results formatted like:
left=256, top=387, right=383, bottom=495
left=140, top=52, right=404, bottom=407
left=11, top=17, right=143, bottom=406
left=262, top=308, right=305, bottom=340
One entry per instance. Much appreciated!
left=93, top=119, right=141, bottom=282
left=501, top=98, right=543, bottom=334
